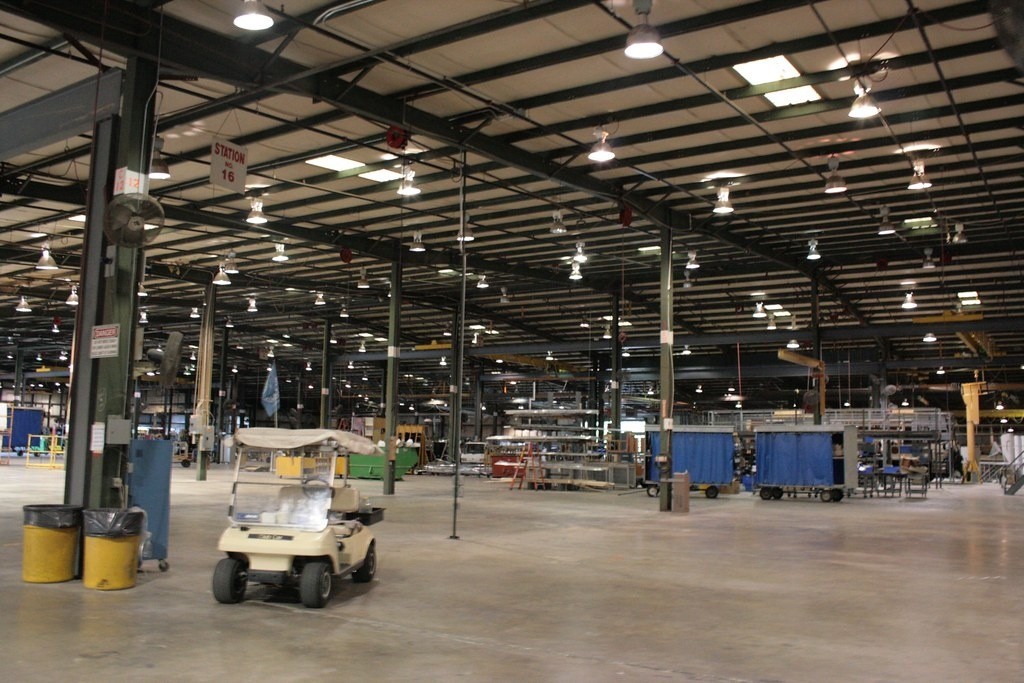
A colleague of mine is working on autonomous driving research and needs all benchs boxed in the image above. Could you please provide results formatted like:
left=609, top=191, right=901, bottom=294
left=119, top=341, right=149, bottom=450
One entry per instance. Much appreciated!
left=276, top=485, right=359, bottom=535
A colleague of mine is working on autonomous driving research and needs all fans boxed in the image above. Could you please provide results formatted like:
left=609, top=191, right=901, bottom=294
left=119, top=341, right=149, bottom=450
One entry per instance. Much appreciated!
left=141, top=331, right=184, bottom=391
left=102, top=192, right=166, bottom=248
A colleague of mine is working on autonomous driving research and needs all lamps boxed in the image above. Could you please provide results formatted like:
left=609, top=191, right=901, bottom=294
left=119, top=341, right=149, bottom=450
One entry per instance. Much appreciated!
left=848, top=74, right=882, bottom=118
left=232, top=0, right=275, bottom=31
left=13, top=249, right=79, bottom=361
left=624, top=0, right=664, bottom=61
left=135, top=129, right=1006, bottom=412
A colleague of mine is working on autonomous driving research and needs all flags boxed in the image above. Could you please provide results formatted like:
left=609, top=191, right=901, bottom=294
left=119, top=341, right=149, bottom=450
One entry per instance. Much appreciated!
left=262, top=362, right=279, bottom=416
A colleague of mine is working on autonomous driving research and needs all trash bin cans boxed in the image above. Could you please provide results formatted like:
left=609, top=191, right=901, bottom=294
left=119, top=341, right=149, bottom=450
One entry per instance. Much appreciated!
left=22, top=504, right=82, bottom=583
left=82, top=509, right=144, bottom=590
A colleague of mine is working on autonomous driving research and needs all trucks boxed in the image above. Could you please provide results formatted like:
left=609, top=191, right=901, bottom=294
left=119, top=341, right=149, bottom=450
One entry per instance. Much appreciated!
left=460, top=442, right=489, bottom=463
